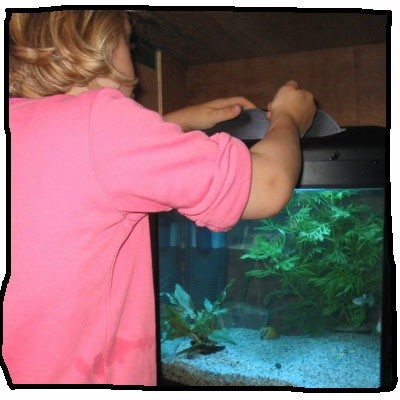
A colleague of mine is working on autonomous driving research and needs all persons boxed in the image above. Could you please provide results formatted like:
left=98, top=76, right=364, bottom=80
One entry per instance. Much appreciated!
left=2, top=9, right=316, bottom=386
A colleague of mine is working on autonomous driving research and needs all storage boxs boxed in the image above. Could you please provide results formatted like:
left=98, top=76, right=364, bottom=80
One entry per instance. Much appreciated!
left=155, top=125, right=388, bottom=389
